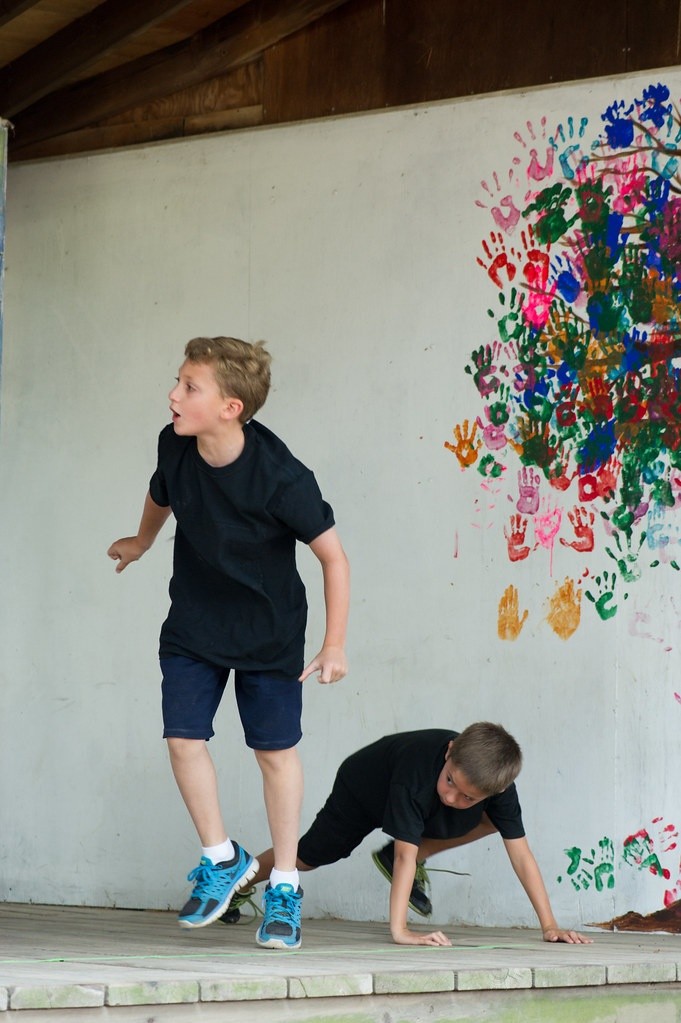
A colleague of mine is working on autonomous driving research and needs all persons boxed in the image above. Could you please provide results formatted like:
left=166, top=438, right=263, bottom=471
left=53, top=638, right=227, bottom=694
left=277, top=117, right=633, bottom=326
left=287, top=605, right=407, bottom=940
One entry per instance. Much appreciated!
left=208, top=721, right=591, bottom=957
left=106, top=333, right=353, bottom=950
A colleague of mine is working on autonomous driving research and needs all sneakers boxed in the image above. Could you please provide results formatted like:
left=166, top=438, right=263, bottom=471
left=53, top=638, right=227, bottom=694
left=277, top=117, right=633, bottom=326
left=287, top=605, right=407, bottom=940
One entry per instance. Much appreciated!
left=178, top=841, right=261, bottom=929
left=256, top=883, right=303, bottom=948
left=372, top=840, right=432, bottom=917
left=218, top=892, right=253, bottom=924
